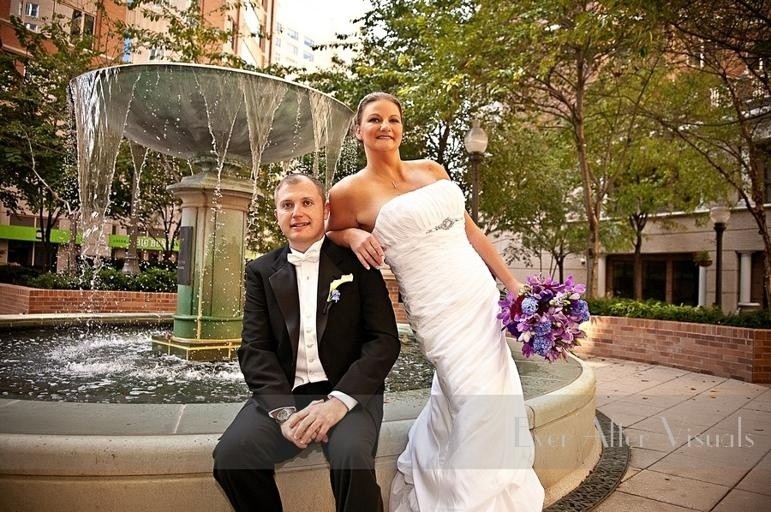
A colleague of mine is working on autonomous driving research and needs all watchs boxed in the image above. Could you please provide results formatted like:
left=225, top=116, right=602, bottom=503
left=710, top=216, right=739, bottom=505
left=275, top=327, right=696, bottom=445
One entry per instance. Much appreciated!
left=273, top=406, right=293, bottom=424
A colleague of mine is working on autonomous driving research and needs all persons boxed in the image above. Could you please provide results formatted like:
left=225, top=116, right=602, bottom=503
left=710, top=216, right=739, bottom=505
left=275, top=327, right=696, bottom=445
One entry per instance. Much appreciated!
left=210, top=173, right=399, bottom=511
left=322, top=91, right=547, bottom=512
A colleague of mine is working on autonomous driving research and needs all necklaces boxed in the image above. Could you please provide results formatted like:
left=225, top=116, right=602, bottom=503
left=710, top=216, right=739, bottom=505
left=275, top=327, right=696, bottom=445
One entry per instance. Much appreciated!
left=365, top=157, right=403, bottom=192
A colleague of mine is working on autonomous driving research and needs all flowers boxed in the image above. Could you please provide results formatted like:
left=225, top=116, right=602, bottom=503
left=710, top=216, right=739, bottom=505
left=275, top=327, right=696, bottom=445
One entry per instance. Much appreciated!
left=496, top=273, right=593, bottom=364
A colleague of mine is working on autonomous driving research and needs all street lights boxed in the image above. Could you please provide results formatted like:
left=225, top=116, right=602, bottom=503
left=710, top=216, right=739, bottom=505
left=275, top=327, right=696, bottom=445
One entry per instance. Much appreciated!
left=463, top=116, right=488, bottom=228
left=709, top=194, right=730, bottom=309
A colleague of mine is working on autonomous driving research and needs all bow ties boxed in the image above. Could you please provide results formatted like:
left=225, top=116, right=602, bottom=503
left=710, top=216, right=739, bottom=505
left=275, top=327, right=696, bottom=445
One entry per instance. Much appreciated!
left=286, top=249, right=319, bottom=267
